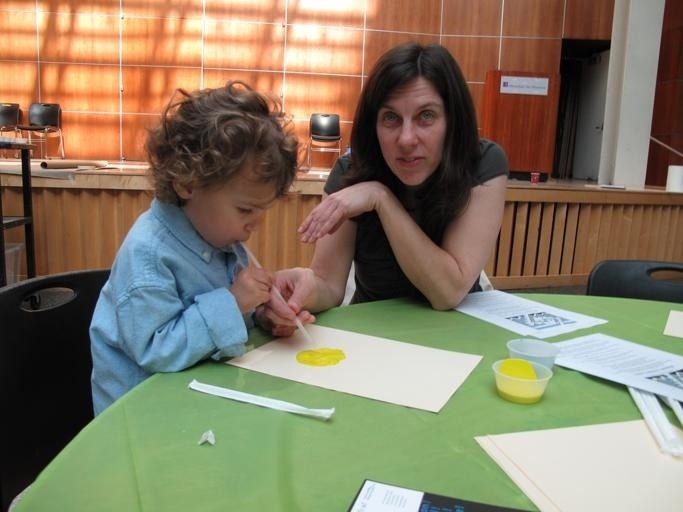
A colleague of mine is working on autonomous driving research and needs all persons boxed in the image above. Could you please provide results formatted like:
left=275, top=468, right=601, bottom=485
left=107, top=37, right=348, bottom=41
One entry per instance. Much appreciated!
left=85, top=81, right=315, bottom=430
left=254, top=40, right=508, bottom=338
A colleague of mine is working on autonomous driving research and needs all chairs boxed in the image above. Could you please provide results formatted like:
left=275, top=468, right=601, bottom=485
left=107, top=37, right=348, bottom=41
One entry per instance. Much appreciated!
left=0, top=102, right=65, bottom=161
left=586, top=258, right=683, bottom=303
left=0, top=268, right=114, bottom=512
left=305, top=112, right=341, bottom=168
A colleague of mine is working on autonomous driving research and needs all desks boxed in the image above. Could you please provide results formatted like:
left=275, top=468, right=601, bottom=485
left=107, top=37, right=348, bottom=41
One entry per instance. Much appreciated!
left=12, top=291, right=683, bottom=512
